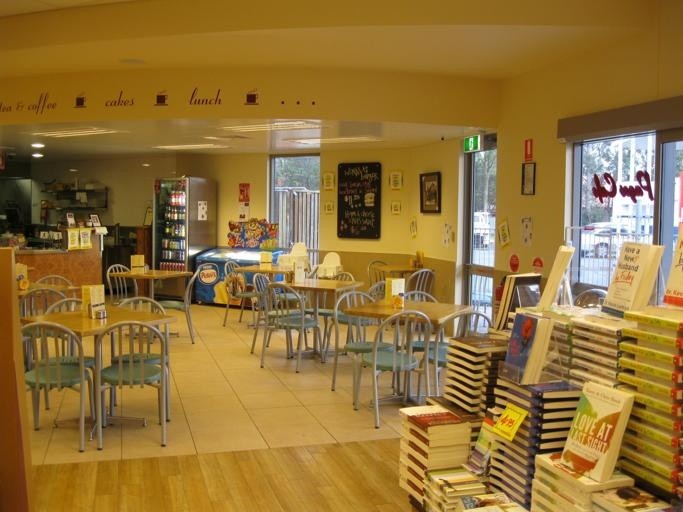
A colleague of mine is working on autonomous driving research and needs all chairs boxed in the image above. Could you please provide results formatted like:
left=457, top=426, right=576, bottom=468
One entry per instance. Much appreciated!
left=22, top=321, right=99, bottom=451
left=95, top=319, right=168, bottom=447
left=411, top=311, right=495, bottom=403
left=354, top=310, right=433, bottom=428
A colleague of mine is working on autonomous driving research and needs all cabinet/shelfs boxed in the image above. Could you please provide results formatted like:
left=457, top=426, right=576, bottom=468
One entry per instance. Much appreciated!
left=39, top=188, right=111, bottom=212
left=103, top=225, right=152, bottom=298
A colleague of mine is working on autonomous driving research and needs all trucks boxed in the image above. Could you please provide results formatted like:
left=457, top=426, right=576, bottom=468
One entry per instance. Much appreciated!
left=611, top=181, right=682, bottom=239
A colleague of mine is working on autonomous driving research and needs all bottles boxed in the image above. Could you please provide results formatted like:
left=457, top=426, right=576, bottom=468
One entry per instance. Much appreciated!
left=163, top=207, right=185, bottom=221
left=160, top=238, right=185, bottom=250
left=96, top=309, right=107, bottom=318
left=165, top=191, right=184, bottom=206
left=162, top=222, right=184, bottom=237
left=397, top=292, right=404, bottom=308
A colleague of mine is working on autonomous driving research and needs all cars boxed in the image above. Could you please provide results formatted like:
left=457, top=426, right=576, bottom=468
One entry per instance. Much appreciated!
left=580, top=222, right=653, bottom=260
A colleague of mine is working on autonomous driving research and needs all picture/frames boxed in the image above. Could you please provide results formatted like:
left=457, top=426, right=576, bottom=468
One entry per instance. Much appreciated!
left=419, top=171, right=441, bottom=214
left=520, top=162, right=536, bottom=195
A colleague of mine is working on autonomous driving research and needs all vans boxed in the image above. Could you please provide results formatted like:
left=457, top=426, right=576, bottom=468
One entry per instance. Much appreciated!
left=473, top=211, right=491, bottom=246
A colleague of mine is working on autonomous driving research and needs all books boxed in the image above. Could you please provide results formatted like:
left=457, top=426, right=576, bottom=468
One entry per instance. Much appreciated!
left=397, top=272, right=683, bottom=512
left=535, top=245, right=567, bottom=312
left=544, top=247, right=575, bottom=313
left=600, top=242, right=664, bottom=318
left=661, top=221, right=683, bottom=310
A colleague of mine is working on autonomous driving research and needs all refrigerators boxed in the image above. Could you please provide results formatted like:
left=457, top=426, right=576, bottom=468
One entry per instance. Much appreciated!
left=151, top=175, right=218, bottom=304
left=193, top=246, right=288, bottom=307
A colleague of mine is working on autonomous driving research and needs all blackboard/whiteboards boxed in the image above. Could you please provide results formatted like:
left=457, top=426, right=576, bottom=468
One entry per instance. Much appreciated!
left=337, top=163, right=380, bottom=239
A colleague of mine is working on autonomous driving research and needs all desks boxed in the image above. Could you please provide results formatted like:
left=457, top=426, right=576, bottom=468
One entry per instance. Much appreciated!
left=344, top=301, right=471, bottom=405
left=22, top=305, right=177, bottom=428
